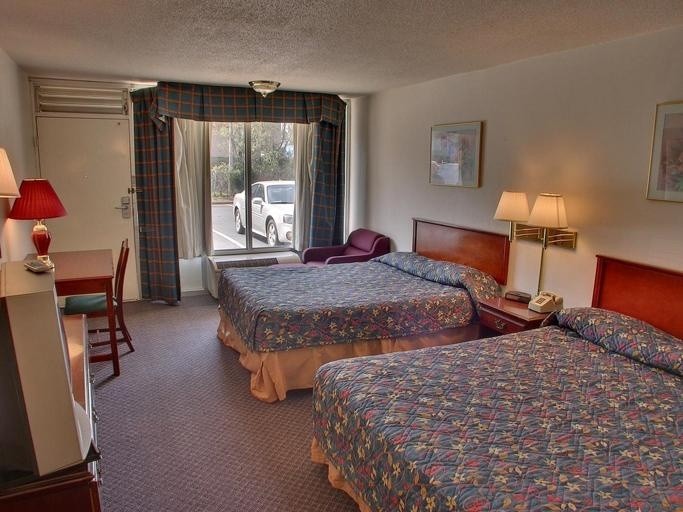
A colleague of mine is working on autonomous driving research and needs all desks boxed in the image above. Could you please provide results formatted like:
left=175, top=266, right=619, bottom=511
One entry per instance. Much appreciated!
left=0, top=314, right=104, bottom=511
left=24, top=249, right=120, bottom=377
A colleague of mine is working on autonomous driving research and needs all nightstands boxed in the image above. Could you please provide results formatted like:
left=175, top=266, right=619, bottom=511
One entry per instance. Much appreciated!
left=476, top=297, right=555, bottom=339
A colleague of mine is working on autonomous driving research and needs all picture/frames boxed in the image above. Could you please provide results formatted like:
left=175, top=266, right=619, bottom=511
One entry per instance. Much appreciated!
left=644, top=100, right=683, bottom=203
left=428, top=121, right=482, bottom=189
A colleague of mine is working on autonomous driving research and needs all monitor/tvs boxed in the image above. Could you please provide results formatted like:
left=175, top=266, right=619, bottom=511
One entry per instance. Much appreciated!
left=0, top=261, right=93, bottom=478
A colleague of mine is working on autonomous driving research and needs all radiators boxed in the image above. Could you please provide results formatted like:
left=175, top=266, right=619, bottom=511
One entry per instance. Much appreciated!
left=207, top=251, right=301, bottom=300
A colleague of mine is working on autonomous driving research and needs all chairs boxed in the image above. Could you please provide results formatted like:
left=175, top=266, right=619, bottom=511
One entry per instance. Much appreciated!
left=302, top=228, right=390, bottom=268
left=64, top=238, right=135, bottom=352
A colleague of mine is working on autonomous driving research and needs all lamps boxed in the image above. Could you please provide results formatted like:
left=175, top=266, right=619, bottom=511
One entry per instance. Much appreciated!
left=492, top=191, right=540, bottom=243
left=249, top=80, right=281, bottom=98
left=0, top=147, right=21, bottom=199
left=8, top=178, right=69, bottom=255
left=526, top=192, right=577, bottom=250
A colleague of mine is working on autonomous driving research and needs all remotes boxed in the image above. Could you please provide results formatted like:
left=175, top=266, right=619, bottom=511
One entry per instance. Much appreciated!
left=24, top=261, right=52, bottom=273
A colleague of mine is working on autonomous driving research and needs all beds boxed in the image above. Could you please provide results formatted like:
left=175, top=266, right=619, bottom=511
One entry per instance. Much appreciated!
left=216, top=218, right=510, bottom=402
left=311, top=254, right=683, bottom=511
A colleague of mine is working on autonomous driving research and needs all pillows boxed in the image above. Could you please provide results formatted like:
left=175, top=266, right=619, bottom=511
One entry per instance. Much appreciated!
left=558, top=309, right=682, bottom=379
left=380, top=251, right=494, bottom=292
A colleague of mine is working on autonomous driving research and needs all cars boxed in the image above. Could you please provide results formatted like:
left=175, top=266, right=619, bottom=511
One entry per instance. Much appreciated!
left=232, top=180, right=294, bottom=247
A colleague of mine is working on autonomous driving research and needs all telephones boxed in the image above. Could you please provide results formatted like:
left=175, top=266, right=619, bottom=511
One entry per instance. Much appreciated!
left=527, top=290, right=563, bottom=314
